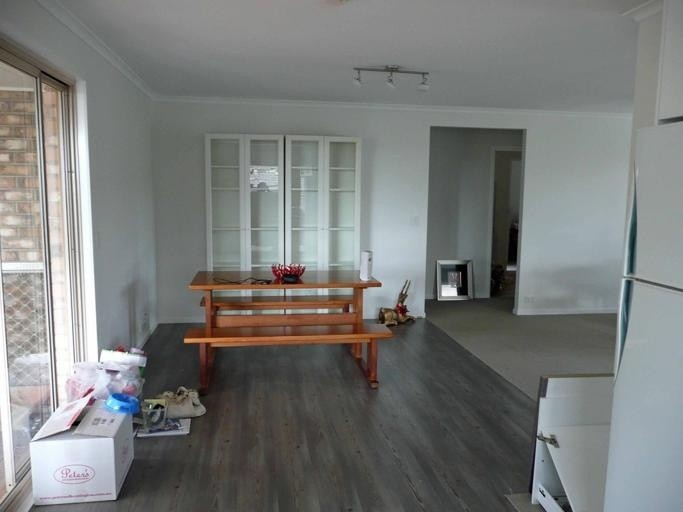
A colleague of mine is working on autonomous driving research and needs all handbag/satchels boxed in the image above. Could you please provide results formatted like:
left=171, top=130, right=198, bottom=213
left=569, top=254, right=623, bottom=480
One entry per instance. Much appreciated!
left=157, top=386, right=206, bottom=418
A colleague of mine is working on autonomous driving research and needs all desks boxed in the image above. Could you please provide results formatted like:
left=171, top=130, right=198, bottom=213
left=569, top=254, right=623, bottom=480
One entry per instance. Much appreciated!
left=188, top=270, right=381, bottom=358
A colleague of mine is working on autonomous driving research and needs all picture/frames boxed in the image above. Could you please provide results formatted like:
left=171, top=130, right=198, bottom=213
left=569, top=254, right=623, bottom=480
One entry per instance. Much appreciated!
left=436, top=259, right=473, bottom=301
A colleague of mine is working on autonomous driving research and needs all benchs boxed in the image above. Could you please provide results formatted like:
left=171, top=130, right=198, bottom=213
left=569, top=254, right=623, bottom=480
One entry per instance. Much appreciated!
left=183, top=324, right=393, bottom=395
left=200, top=296, right=354, bottom=315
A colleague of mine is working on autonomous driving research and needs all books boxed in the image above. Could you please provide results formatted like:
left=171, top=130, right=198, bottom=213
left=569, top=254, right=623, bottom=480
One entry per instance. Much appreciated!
left=136, top=417, right=192, bottom=438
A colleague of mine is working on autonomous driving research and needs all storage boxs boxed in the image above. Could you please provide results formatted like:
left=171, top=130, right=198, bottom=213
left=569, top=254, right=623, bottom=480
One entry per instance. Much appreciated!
left=28, top=392, right=134, bottom=506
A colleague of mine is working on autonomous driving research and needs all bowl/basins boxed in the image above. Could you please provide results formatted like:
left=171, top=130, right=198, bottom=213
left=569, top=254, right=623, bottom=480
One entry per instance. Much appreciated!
left=106, top=393, right=141, bottom=415
left=140, top=404, right=168, bottom=430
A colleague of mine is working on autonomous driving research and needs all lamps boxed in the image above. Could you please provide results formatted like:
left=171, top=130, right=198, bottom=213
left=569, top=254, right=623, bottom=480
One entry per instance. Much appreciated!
left=352, top=65, right=431, bottom=90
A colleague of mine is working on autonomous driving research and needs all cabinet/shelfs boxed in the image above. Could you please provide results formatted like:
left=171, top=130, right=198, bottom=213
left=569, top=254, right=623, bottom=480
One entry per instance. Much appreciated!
left=285, top=135, right=362, bottom=314
left=205, top=133, right=284, bottom=315
